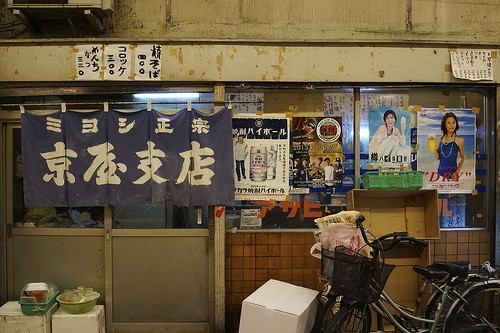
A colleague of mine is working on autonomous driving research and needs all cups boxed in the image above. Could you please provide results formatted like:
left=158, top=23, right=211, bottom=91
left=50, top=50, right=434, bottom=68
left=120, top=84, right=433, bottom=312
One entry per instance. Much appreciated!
left=428, top=133, right=436, bottom=152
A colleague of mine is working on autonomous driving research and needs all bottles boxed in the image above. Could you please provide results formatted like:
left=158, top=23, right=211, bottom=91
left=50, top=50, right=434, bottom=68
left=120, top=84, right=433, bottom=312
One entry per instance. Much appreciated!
left=401, top=116, right=406, bottom=130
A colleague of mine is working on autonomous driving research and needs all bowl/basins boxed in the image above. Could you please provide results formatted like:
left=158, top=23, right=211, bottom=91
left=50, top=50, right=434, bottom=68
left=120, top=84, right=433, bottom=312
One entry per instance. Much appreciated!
left=25, top=283, right=49, bottom=292
left=56, top=290, right=100, bottom=314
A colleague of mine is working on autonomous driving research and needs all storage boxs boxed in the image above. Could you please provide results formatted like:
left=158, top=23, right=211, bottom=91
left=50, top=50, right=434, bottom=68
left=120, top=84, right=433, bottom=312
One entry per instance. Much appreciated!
left=51, top=305, right=106, bottom=333
left=347, top=189, right=440, bottom=241
left=371, top=242, right=431, bottom=332
left=238, top=279, right=320, bottom=333
left=0, top=301, right=58, bottom=333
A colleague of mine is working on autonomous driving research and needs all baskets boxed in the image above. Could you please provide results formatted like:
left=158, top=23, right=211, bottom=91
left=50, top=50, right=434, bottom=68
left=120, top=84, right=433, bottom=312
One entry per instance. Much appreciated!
left=332, top=245, right=396, bottom=305
left=320, top=245, right=374, bottom=289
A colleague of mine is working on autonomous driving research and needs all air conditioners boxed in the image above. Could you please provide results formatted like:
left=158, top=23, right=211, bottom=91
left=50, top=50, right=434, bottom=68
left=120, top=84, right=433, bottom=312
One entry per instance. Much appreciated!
left=7, top=0, right=114, bottom=23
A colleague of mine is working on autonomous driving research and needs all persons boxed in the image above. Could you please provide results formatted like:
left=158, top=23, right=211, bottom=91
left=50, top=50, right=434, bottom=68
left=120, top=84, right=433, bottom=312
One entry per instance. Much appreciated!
left=294, top=157, right=343, bottom=188
left=233, top=135, right=247, bottom=183
left=367, top=110, right=407, bottom=162
left=431, top=112, right=466, bottom=182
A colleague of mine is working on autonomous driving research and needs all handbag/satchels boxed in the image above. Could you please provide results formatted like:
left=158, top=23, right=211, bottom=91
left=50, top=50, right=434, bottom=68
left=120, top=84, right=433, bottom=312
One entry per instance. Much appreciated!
left=311, top=211, right=374, bottom=279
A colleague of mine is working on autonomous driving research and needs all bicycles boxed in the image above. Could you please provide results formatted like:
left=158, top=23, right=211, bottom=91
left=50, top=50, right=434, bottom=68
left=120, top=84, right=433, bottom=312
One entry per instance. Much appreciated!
left=310, top=214, right=500, bottom=333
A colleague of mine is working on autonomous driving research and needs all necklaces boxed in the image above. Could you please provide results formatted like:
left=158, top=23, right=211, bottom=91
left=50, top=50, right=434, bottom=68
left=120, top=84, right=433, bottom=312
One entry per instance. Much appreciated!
left=440, top=132, right=456, bottom=157
